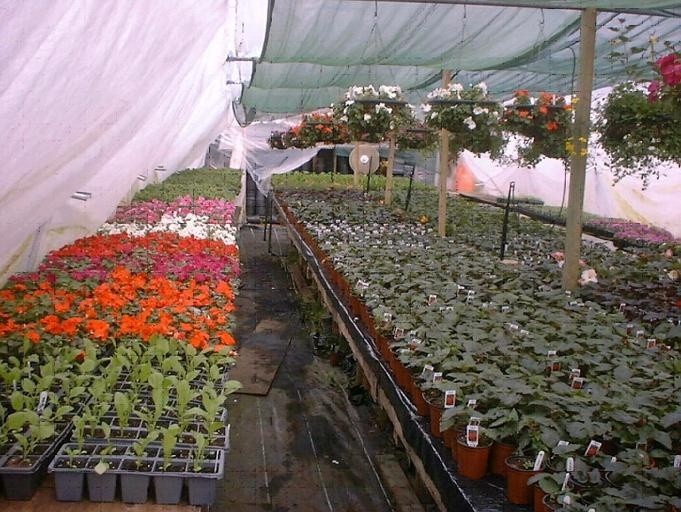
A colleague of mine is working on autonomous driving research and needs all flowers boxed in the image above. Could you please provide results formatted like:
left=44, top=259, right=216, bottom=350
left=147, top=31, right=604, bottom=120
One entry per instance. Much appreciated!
left=640, top=39, right=681, bottom=108
left=0, top=192, right=242, bottom=356
left=291, top=81, right=590, bottom=158
